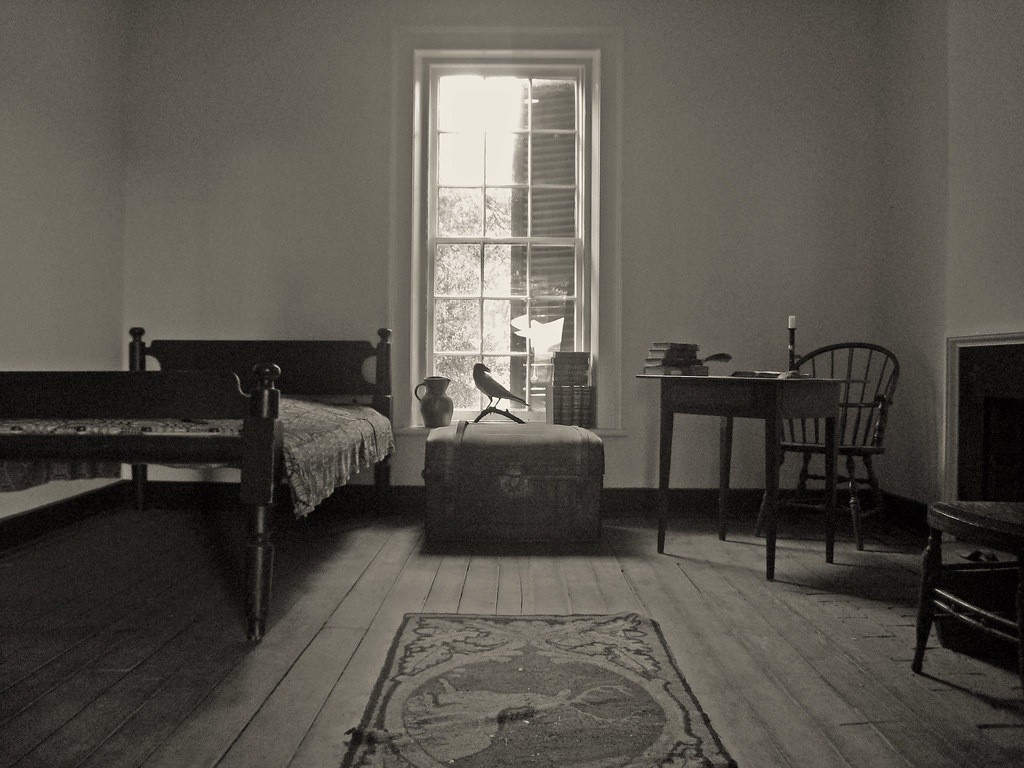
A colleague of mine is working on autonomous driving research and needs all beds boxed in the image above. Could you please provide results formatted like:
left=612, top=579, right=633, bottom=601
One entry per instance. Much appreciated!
left=0, top=326, right=395, bottom=646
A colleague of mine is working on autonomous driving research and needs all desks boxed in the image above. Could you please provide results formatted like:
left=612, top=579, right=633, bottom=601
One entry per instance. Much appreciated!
left=636, top=375, right=870, bottom=581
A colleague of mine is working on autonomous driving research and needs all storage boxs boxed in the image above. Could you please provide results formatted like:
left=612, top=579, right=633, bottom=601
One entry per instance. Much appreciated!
left=421, top=420, right=606, bottom=553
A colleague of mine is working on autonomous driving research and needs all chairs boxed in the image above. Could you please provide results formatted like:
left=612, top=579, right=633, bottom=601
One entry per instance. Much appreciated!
left=754, top=341, right=900, bottom=551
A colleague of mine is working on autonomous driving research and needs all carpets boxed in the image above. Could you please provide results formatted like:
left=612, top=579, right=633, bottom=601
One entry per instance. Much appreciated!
left=340, top=612, right=738, bottom=768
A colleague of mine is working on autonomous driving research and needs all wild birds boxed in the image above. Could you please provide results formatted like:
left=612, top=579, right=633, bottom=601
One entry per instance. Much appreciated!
left=473, top=362, right=530, bottom=410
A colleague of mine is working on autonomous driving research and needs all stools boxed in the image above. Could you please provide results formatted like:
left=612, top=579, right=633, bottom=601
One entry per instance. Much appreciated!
left=911, top=500, right=1024, bottom=681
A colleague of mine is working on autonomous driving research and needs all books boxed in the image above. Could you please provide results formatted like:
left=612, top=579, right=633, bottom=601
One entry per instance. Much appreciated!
left=643, top=342, right=710, bottom=375
left=549, top=351, right=591, bottom=387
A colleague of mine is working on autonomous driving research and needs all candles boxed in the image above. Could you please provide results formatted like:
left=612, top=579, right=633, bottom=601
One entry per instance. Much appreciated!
left=788, top=316, right=795, bottom=328
